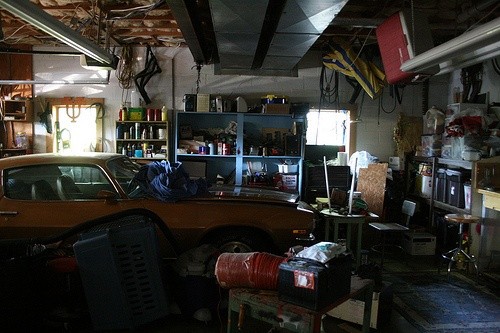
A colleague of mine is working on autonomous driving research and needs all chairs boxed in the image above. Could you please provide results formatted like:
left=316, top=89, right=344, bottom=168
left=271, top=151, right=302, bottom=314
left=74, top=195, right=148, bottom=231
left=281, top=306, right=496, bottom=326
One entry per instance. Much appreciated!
left=31, top=180, right=53, bottom=200
left=369, top=199, right=417, bottom=269
left=56, top=175, right=82, bottom=199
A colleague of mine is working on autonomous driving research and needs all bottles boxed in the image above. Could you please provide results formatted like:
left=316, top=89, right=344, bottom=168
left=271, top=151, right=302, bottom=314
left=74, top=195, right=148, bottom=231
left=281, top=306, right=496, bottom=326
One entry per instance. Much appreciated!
left=116, top=103, right=168, bottom=163
left=200, top=137, right=284, bottom=157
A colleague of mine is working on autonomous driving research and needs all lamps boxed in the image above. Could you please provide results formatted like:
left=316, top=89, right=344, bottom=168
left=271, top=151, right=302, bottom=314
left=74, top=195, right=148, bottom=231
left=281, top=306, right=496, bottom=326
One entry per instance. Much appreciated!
left=399, top=0, right=500, bottom=73
left=0, top=0, right=120, bottom=71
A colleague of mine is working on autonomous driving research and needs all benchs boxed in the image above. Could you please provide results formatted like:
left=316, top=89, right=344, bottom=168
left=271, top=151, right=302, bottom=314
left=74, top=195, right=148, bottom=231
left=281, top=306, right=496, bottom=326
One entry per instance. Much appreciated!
left=6, top=179, right=28, bottom=198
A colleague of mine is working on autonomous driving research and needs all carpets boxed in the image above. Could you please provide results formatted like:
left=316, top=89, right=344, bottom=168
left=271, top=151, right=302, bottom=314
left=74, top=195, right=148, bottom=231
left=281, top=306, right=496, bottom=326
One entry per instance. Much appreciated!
left=385, top=274, right=500, bottom=333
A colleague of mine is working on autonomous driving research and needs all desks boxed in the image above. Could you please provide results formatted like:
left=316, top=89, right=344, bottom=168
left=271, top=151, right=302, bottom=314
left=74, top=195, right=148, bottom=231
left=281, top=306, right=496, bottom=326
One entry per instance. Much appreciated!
left=324, top=215, right=368, bottom=265
left=227, top=278, right=375, bottom=333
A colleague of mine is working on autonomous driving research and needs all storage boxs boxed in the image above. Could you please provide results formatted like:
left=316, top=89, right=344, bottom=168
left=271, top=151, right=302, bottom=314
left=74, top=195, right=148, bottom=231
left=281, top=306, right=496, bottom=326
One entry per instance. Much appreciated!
left=265, top=104, right=291, bottom=114
left=196, top=94, right=209, bottom=112
left=376, top=10, right=434, bottom=85
left=182, top=162, right=206, bottom=176
left=274, top=173, right=298, bottom=191
left=390, top=91, right=489, bottom=168
left=278, top=164, right=298, bottom=173
left=404, top=232, right=437, bottom=256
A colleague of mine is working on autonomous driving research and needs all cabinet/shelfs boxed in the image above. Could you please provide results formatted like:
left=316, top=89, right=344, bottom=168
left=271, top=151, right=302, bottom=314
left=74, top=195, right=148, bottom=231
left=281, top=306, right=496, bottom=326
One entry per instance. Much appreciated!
left=390, top=155, right=500, bottom=289
left=174, top=110, right=305, bottom=195
left=0, top=53, right=33, bottom=156
left=115, top=120, right=169, bottom=162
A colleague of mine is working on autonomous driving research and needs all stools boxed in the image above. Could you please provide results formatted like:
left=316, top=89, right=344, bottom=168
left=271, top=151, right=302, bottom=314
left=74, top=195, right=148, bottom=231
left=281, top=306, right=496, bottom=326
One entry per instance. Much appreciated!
left=443, top=213, right=481, bottom=282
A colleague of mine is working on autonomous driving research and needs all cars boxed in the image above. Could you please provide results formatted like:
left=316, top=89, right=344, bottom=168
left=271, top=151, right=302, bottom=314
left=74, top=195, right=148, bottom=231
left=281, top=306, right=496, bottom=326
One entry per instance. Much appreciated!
left=1, top=151, right=323, bottom=301
